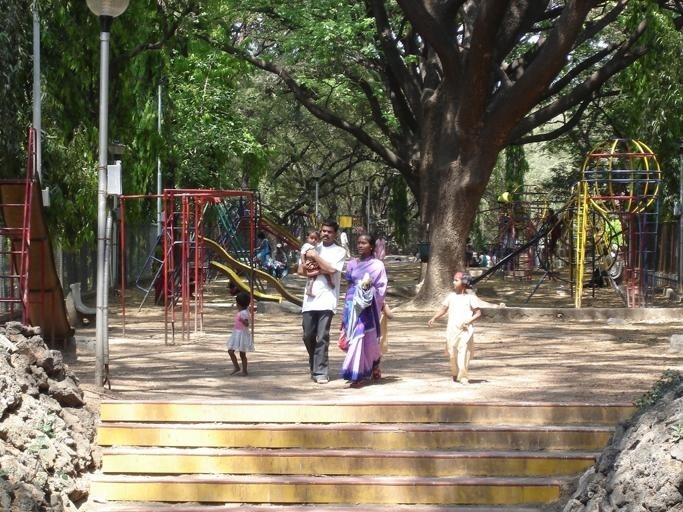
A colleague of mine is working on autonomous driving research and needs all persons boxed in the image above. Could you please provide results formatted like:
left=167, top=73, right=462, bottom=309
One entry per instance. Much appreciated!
left=297, top=220, right=394, bottom=389
left=427, top=271, right=482, bottom=385
left=226, top=291, right=256, bottom=377
left=465, top=236, right=473, bottom=268
left=545, top=207, right=562, bottom=251
left=253, top=231, right=288, bottom=283
left=596, top=225, right=607, bottom=255
left=585, top=243, right=622, bottom=287
left=152, top=235, right=172, bottom=308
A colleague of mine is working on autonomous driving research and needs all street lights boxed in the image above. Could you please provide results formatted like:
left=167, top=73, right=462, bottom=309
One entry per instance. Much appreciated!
left=85, top=0, right=130, bottom=387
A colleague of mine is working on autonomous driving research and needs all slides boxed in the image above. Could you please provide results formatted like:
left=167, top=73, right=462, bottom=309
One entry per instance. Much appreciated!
left=209, top=261, right=282, bottom=303
left=198, top=236, right=303, bottom=306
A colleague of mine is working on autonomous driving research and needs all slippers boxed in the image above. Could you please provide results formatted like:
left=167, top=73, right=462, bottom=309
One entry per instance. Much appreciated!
left=350, top=381, right=366, bottom=387
left=316, top=378, right=329, bottom=384
left=373, top=368, right=381, bottom=381
